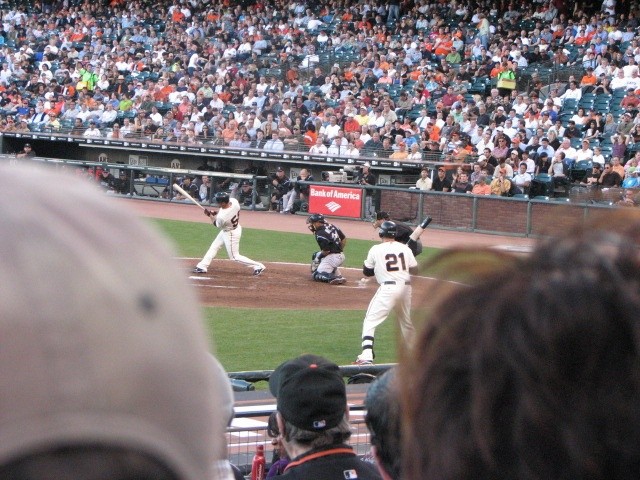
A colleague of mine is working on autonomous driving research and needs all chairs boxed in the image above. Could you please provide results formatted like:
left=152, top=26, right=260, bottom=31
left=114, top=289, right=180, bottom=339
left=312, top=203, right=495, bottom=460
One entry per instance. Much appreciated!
left=124, top=70, right=159, bottom=84
left=522, top=62, right=564, bottom=82
left=558, top=90, right=628, bottom=124
left=566, top=42, right=586, bottom=55
left=459, top=73, right=497, bottom=97
left=146, top=177, right=170, bottom=186
left=143, top=22, right=160, bottom=38
left=72, top=40, right=86, bottom=48
left=556, top=130, right=619, bottom=158
left=221, top=110, right=230, bottom=118
left=406, top=104, right=441, bottom=120
left=99, top=32, right=118, bottom=41
left=509, top=175, right=612, bottom=209
left=258, top=68, right=282, bottom=77
left=325, top=98, right=335, bottom=106
left=314, top=49, right=362, bottom=64
left=312, top=14, right=341, bottom=38
left=386, top=15, right=546, bottom=47
left=27, top=99, right=174, bottom=135
left=374, top=81, right=419, bottom=103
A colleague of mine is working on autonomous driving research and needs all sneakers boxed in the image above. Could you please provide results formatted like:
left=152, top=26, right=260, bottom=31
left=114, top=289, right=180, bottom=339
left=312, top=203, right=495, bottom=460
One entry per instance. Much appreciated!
left=356, top=351, right=373, bottom=364
left=329, top=275, right=347, bottom=285
left=193, top=266, right=207, bottom=273
left=253, top=265, right=266, bottom=276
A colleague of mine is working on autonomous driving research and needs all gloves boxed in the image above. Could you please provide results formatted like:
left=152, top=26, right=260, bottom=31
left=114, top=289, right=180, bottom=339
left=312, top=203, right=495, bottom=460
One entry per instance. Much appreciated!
left=204, top=209, right=210, bottom=217
left=210, top=211, right=217, bottom=216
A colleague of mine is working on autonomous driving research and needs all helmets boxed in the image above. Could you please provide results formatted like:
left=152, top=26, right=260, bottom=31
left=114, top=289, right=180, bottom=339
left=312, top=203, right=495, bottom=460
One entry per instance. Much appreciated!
left=379, top=220, right=397, bottom=238
left=211, top=192, right=229, bottom=204
left=306, top=213, right=328, bottom=233
left=369, top=212, right=390, bottom=229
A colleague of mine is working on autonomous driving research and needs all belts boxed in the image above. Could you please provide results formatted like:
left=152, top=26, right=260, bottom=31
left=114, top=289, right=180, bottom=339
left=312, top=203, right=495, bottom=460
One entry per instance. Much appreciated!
left=384, top=281, right=410, bottom=285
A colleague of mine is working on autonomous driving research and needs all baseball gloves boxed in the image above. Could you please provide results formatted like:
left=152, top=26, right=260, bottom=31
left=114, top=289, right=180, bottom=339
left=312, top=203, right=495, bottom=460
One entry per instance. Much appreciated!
left=313, top=252, right=324, bottom=264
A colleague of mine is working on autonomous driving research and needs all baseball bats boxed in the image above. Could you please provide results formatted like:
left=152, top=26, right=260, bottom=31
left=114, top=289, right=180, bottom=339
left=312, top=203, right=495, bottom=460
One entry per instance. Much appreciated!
left=173, top=183, right=206, bottom=211
left=406, top=216, right=433, bottom=247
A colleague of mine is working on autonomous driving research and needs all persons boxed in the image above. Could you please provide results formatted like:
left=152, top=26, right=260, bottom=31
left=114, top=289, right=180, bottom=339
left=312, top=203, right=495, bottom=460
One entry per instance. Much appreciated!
left=525, top=91, right=544, bottom=112
left=609, top=132, right=630, bottom=158
left=523, top=104, right=541, bottom=123
left=535, top=113, right=553, bottom=129
left=450, top=167, right=465, bottom=188
left=365, top=368, right=402, bottom=479
left=632, top=112, right=640, bottom=124
left=198, top=175, right=211, bottom=200
left=453, top=0, right=504, bottom=163
left=285, top=0, right=323, bottom=155
left=506, top=138, right=524, bottom=160
left=16, top=143, right=36, bottom=157
left=239, top=180, right=262, bottom=205
left=504, top=119, right=518, bottom=140
left=34, top=0, right=61, bottom=133
left=415, top=167, right=432, bottom=189
left=505, top=96, right=512, bottom=113
left=546, top=152, right=569, bottom=200
left=271, top=167, right=297, bottom=213
left=605, top=157, right=624, bottom=178
left=624, top=151, right=640, bottom=168
left=604, top=113, right=617, bottom=134
left=126, top=0, right=152, bottom=141
left=546, top=130, right=560, bottom=150
left=99, top=167, right=116, bottom=184
left=595, top=112, right=604, bottom=135
left=629, top=159, right=638, bottom=169
left=619, top=88, right=640, bottom=108
left=113, top=172, right=137, bottom=195
left=367, top=2, right=409, bottom=160
left=373, top=213, right=422, bottom=256
left=544, top=89, right=561, bottom=110
left=103, top=0, right=126, bottom=141
left=189, top=192, right=267, bottom=278
left=592, top=78, right=613, bottom=95
left=598, top=163, right=623, bottom=186
left=471, top=164, right=481, bottom=180
left=492, top=158, right=513, bottom=179
left=629, top=124, right=640, bottom=144
left=618, top=113, right=634, bottom=132
left=359, top=165, right=376, bottom=219
left=395, top=188, right=636, bottom=480
left=525, top=136, right=541, bottom=152
left=528, top=128, right=546, bottom=145
left=83, top=0, right=103, bottom=139
left=174, top=177, right=197, bottom=199
left=568, top=107, right=588, bottom=125
left=197, top=158, right=215, bottom=170
left=216, top=159, right=233, bottom=171
left=505, top=150, right=520, bottom=169
left=409, top=1, right=453, bottom=162
left=355, top=221, right=418, bottom=363
left=588, top=109, right=596, bottom=120
left=519, top=151, right=535, bottom=173
left=251, top=0, right=285, bottom=151
left=592, top=146, right=606, bottom=163
left=491, top=169, right=511, bottom=194
left=580, top=162, right=604, bottom=187
left=0, top=0, right=11, bottom=132
left=511, top=163, right=532, bottom=193
left=514, top=133, right=526, bottom=151
left=268, top=410, right=291, bottom=478
left=217, top=0, right=251, bottom=149
left=453, top=172, right=473, bottom=194
left=538, top=138, right=555, bottom=156
left=548, top=119, right=565, bottom=138
left=11, top=1, right=34, bottom=132
left=504, top=2, right=640, bottom=88
left=307, top=214, right=346, bottom=285
left=583, top=121, right=597, bottom=139
left=152, top=0, right=181, bottom=145
left=559, top=81, right=583, bottom=100
left=622, top=103, right=639, bottom=118
left=512, top=89, right=519, bottom=100
left=281, top=168, right=315, bottom=213
left=323, top=1, right=367, bottom=157
left=536, top=152, right=552, bottom=174
left=509, top=108, right=520, bottom=120
left=430, top=166, right=452, bottom=191
left=539, top=99, right=558, bottom=122
left=269, top=353, right=382, bottom=480
left=61, top=0, right=83, bottom=136
left=476, top=168, right=490, bottom=181
left=181, top=0, right=217, bottom=146
left=517, top=120, right=532, bottom=139
left=513, top=95, right=528, bottom=115
left=471, top=175, right=491, bottom=195
left=555, top=137, right=577, bottom=159
left=575, top=139, right=593, bottom=162
left=79, top=166, right=93, bottom=181
left=561, top=121, right=582, bottom=137
left=622, top=167, right=640, bottom=188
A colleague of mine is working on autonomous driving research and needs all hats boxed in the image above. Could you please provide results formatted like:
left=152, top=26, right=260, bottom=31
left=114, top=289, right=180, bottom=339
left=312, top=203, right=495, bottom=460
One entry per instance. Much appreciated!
left=268, top=355, right=347, bottom=431
